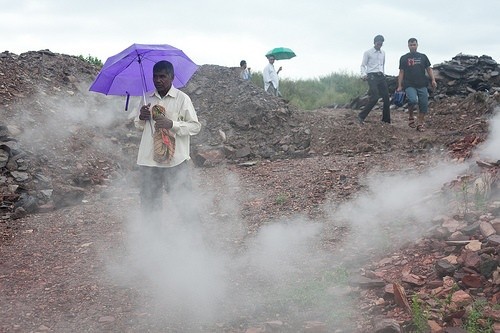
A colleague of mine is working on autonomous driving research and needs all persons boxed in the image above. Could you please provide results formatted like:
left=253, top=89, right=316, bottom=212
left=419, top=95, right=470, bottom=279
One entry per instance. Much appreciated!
left=240, top=60, right=251, bottom=79
left=264, top=55, right=282, bottom=96
left=356, top=34, right=390, bottom=123
left=134, top=61, right=201, bottom=226
left=397, top=38, right=437, bottom=131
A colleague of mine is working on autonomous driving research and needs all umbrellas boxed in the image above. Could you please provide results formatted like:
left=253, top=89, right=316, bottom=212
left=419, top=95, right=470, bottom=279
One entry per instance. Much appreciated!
left=265, top=47, right=296, bottom=60
left=89, top=43, right=199, bottom=106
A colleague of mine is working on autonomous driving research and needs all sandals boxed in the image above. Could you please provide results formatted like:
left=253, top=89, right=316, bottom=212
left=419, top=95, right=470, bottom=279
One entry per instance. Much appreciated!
left=417, top=123, right=425, bottom=132
left=408, top=117, right=416, bottom=128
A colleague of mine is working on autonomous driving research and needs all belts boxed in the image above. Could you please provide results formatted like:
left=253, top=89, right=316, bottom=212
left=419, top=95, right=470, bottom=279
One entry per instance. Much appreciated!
left=372, top=72, right=383, bottom=75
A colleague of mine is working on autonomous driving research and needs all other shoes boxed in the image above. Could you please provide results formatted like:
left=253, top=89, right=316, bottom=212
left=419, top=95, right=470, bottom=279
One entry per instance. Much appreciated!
left=356, top=114, right=366, bottom=124
